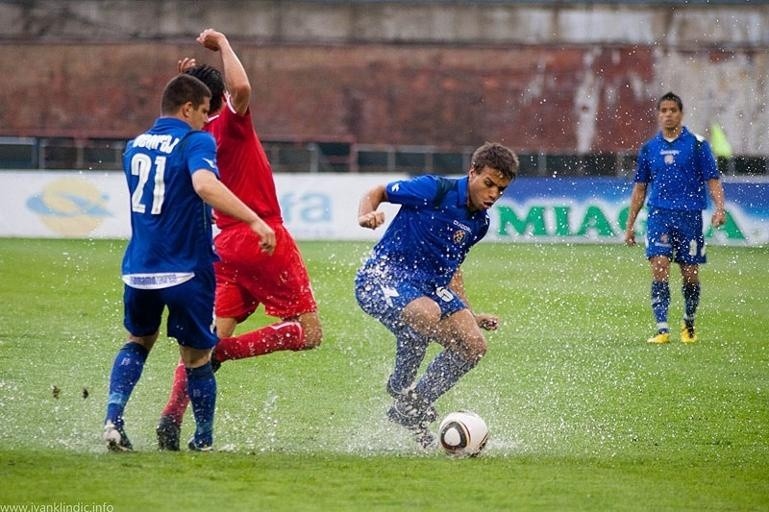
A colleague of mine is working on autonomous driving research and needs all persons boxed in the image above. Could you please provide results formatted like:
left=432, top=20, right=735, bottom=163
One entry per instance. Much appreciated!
left=103, top=75, right=276, bottom=451
left=354, top=141, right=520, bottom=455
left=625, top=93, right=726, bottom=344
left=156, top=28, right=323, bottom=450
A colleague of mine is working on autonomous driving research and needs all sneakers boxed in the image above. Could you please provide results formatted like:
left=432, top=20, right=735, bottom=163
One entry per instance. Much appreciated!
left=647, top=327, right=672, bottom=344
left=680, top=318, right=697, bottom=344
left=102, top=418, right=134, bottom=452
left=385, top=370, right=438, bottom=451
left=154, top=415, right=182, bottom=452
left=187, top=426, right=214, bottom=452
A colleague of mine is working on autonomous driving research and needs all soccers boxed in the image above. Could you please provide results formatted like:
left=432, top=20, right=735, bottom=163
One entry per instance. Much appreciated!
left=438, top=408, right=491, bottom=460
